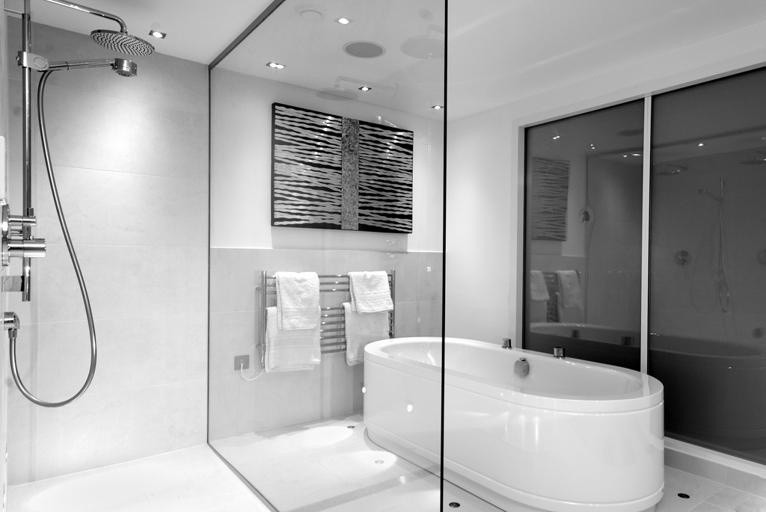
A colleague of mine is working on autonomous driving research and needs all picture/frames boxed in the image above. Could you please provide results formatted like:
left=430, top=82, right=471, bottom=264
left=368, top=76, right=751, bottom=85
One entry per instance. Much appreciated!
left=531, top=159, right=574, bottom=245
left=270, top=103, right=414, bottom=233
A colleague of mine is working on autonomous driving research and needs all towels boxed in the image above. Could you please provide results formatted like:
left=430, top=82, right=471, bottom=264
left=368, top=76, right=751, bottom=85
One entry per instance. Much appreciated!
left=274, top=271, right=322, bottom=329
left=263, top=306, right=322, bottom=375
left=529, top=298, right=546, bottom=324
left=341, top=302, right=390, bottom=367
left=553, top=271, right=582, bottom=309
left=346, top=271, right=395, bottom=313
left=528, top=270, right=550, bottom=301
left=556, top=292, right=583, bottom=326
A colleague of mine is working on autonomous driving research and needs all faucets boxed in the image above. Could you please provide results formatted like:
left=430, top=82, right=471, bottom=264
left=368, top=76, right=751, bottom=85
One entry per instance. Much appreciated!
left=502, top=339, right=511, bottom=348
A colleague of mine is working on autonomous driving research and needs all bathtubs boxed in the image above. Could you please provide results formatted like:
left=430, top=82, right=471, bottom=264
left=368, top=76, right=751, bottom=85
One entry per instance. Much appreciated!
left=363, top=337, right=666, bottom=512
